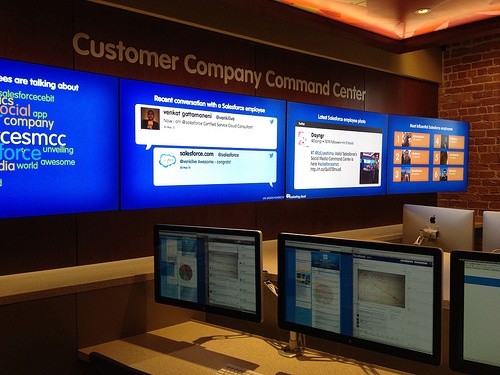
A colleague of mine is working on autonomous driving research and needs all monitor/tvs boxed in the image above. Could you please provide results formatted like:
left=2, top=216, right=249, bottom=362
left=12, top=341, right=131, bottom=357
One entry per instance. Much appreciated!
left=152, top=224, right=264, bottom=323
left=482, top=211, right=500, bottom=253
left=1, top=57, right=473, bottom=219
left=402, top=203, right=474, bottom=251
left=277, top=232, right=443, bottom=366
left=449, top=249, right=500, bottom=375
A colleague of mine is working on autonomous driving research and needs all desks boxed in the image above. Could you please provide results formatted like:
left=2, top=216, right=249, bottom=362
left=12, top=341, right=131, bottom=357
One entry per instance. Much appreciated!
left=81, top=321, right=415, bottom=375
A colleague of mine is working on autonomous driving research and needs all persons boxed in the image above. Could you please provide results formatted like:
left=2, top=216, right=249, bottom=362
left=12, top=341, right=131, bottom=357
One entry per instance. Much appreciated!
left=439, top=135, right=448, bottom=148
left=141, top=110, right=159, bottom=130
left=401, top=150, right=410, bottom=164
left=401, top=132, right=412, bottom=146
left=441, top=169, right=448, bottom=180
left=439, top=152, right=447, bottom=165
left=361, top=153, right=379, bottom=181
left=401, top=170, right=409, bottom=183
